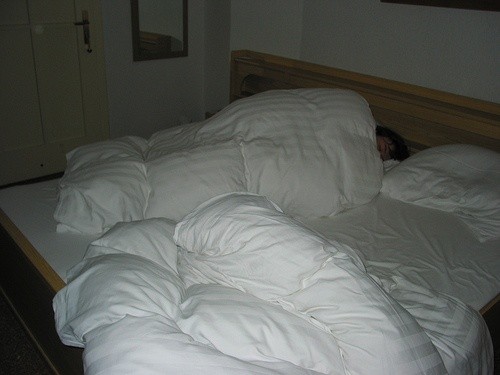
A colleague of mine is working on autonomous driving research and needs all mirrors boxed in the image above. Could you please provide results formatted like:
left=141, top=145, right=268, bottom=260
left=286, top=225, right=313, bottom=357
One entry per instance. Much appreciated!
left=132, top=0, right=189, bottom=62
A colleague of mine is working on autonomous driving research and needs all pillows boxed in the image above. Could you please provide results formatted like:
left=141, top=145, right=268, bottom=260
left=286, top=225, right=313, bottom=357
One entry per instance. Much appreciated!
left=382, top=143, right=500, bottom=243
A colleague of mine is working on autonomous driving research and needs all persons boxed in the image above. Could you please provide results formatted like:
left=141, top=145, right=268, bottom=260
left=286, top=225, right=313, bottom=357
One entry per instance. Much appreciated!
left=375, top=125, right=409, bottom=163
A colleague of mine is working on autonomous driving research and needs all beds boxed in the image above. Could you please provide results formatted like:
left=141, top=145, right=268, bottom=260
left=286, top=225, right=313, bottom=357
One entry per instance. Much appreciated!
left=0, top=51, right=500, bottom=375
